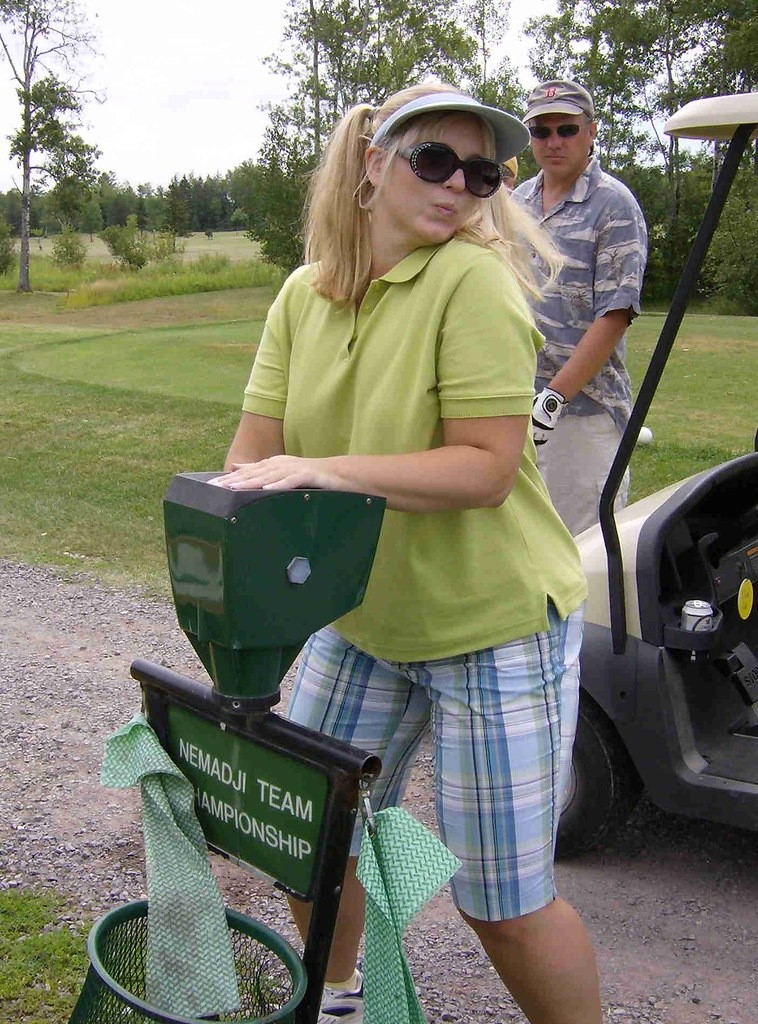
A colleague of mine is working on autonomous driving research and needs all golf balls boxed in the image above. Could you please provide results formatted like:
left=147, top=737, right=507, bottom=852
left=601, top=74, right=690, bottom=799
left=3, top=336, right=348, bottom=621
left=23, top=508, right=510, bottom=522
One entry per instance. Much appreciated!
left=637, top=426, right=653, bottom=447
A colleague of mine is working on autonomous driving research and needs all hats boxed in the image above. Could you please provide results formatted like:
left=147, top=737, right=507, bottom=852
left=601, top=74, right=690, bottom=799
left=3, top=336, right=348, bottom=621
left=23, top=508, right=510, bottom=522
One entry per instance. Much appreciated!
left=368, top=91, right=531, bottom=165
left=522, top=80, right=595, bottom=123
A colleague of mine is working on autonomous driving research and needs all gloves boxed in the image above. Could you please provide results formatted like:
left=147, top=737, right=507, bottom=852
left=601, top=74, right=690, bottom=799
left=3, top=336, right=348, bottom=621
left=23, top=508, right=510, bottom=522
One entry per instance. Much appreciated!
left=529, top=387, right=569, bottom=445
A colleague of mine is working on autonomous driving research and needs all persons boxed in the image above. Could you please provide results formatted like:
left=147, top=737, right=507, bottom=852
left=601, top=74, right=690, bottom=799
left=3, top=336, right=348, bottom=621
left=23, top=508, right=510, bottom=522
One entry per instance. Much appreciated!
left=510, top=81, right=648, bottom=540
left=500, top=156, right=518, bottom=190
left=205, top=81, right=601, bottom=1024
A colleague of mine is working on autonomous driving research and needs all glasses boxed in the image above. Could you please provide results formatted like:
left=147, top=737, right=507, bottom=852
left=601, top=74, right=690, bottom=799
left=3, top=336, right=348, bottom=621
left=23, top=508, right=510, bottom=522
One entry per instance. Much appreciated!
left=384, top=141, right=511, bottom=198
left=529, top=125, right=590, bottom=139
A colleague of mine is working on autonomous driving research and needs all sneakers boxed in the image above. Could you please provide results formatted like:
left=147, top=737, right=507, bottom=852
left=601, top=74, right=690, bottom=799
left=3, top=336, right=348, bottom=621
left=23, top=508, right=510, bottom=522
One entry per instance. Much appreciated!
left=316, top=956, right=365, bottom=1024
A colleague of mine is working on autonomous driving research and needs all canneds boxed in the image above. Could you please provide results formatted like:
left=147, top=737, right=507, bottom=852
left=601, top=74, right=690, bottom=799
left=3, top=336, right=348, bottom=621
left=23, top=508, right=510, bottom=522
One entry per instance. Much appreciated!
left=681, top=600, right=713, bottom=661
